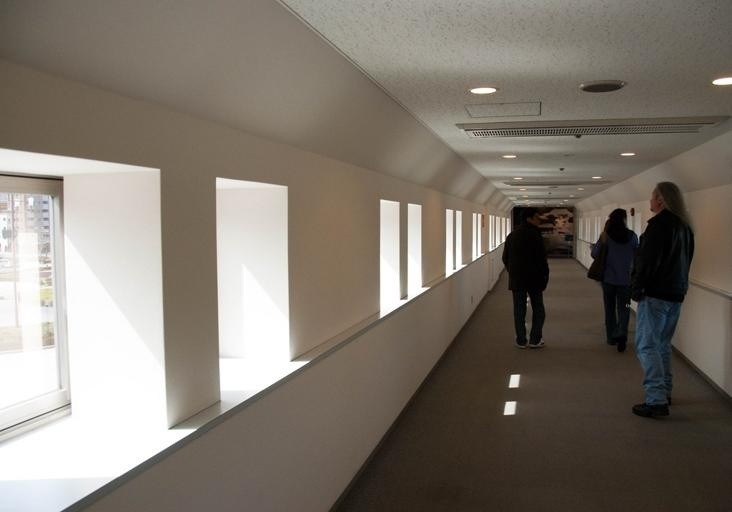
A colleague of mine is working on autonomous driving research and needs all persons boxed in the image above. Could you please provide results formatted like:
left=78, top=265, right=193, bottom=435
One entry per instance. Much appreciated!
left=630, top=183, right=694, bottom=419
left=503, top=207, right=549, bottom=349
left=591, top=209, right=638, bottom=353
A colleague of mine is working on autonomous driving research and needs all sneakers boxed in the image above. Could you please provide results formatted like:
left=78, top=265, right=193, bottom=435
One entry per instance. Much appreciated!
left=528, top=339, right=545, bottom=347
left=632, top=403, right=669, bottom=416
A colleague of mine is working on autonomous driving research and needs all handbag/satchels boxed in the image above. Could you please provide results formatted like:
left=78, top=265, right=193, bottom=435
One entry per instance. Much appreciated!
left=588, top=243, right=606, bottom=281
